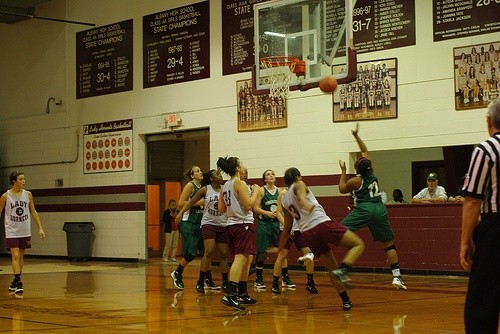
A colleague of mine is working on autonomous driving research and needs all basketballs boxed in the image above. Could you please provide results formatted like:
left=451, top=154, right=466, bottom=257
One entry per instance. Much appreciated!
left=320, top=76, right=337, bottom=93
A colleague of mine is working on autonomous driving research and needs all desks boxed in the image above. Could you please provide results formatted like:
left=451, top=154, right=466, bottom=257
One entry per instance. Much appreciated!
left=350, top=203, right=472, bottom=276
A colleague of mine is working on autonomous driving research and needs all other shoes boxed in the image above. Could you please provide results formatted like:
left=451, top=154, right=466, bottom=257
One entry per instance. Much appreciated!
left=162, top=258, right=169, bottom=262
left=170, top=257, right=176, bottom=262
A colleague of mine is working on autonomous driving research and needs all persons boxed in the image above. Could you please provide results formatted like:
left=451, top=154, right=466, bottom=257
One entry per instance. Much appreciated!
left=296, top=123, right=410, bottom=290
left=0, top=171, right=46, bottom=293
left=170, top=157, right=365, bottom=311
left=411, top=173, right=448, bottom=203
left=459, top=97, right=500, bottom=334
left=386, top=188, right=407, bottom=204
left=449, top=188, right=465, bottom=202
left=163, top=200, right=180, bottom=262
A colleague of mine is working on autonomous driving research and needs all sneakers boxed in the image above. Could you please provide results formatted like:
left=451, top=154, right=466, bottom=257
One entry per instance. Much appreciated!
left=281, top=274, right=297, bottom=287
left=222, top=271, right=228, bottom=287
left=255, top=280, right=266, bottom=288
left=236, top=292, right=257, bottom=305
left=171, top=271, right=184, bottom=289
left=248, top=263, right=256, bottom=277
left=8, top=282, right=16, bottom=291
left=306, top=281, right=318, bottom=294
left=297, top=252, right=315, bottom=265
left=204, top=279, right=221, bottom=289
left=331, top=268, right=352, bottom=283
left=220, top=293, right=246, bottom=311
left=196, top=280, right=205, bottom=292
left=223, top=285, right=237, bottom=295
left=343, top=301, right=353, bottom=310
left=392, top=276, right=407, bottom=290
left=15, top=281, right=24, bottom=293
left=271, top=284, right=281, bottom=294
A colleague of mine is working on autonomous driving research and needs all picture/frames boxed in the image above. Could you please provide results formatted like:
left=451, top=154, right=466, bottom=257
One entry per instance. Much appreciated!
left=331, top=58, right=398, bottom=122
left=236, top=73, right=288, bottom=131
left=453, top=41, right=500, bottom=111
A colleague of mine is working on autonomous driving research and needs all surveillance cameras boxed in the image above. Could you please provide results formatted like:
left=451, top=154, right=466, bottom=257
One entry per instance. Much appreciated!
left=55, top=99, right=60, bottom=105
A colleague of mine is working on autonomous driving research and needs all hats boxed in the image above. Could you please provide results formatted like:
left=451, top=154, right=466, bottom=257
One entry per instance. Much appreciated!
left=427, top=173, right=437, bottom=180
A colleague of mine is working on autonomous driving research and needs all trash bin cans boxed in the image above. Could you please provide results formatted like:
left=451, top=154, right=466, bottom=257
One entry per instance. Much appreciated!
left=61, top=221, right=95, bottom=262
